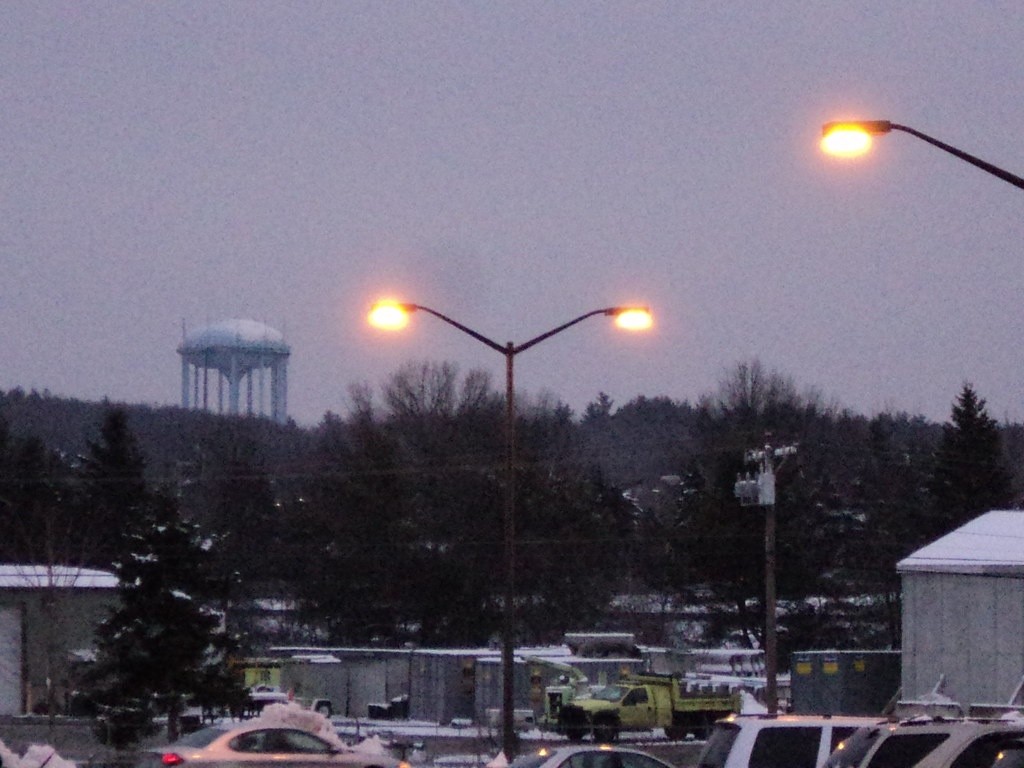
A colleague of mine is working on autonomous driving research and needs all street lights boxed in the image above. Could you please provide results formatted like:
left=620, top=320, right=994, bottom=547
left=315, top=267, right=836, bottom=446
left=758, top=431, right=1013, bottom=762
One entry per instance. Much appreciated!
left=367, top=299, right=653, bottom=761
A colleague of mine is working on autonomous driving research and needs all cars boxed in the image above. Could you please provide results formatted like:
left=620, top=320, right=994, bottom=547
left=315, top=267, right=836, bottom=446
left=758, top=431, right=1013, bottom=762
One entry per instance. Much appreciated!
left=138, top=718, right=411, bottom=768
left=249, top=692, right=332, bottom=718
left=508, top=743, right=675, bottom=768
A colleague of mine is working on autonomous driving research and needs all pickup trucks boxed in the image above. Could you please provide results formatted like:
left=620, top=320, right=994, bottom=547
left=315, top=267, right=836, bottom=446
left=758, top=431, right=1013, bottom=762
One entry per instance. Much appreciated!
left=556, top=670, right=744, bottom=741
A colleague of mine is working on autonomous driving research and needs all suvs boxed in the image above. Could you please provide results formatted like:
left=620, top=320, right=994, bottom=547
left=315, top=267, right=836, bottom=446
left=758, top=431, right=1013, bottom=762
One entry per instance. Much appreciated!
left=820, top=699, right=1024, bottom=768
left=695, top=711, right=888, bottom=768
